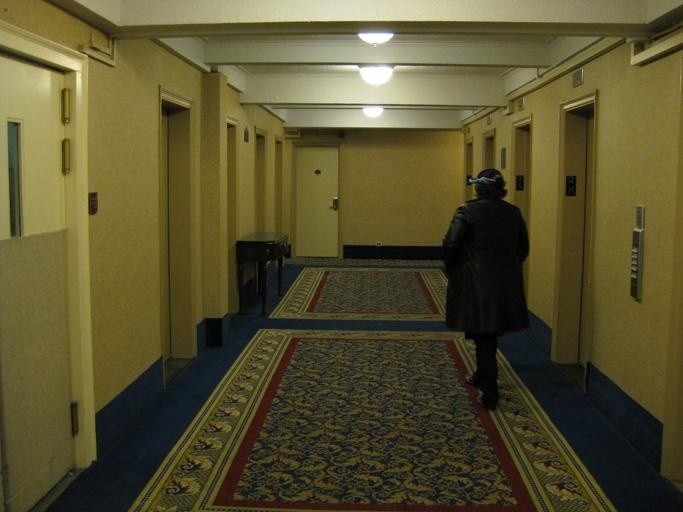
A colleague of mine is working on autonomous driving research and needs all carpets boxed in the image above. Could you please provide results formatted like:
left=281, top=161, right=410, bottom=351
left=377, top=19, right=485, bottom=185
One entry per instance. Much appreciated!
left=268, top=266, right=448, bottom=321
left=127, top=329, right=618, bottom=512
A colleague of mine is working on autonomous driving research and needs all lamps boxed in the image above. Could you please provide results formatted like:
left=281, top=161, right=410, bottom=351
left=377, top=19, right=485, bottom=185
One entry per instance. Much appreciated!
left=358, top=63, right=396, bottom=88
left=358, top=32, right=394, bottom=47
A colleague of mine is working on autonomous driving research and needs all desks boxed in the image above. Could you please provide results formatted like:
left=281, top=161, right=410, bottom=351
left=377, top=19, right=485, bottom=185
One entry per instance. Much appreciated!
left=236, top=232, right=290, bottom=316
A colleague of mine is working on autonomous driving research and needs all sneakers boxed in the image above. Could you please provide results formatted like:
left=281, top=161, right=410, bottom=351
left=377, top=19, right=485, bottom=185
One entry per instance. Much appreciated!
left=466, top=373, right=479, bottom=387
left=477, top=394, right=495, bottom=409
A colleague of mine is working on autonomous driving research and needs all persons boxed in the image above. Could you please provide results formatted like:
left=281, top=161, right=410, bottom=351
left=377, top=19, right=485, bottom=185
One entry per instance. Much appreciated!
left=443, top=168, right=530, bottom=410
